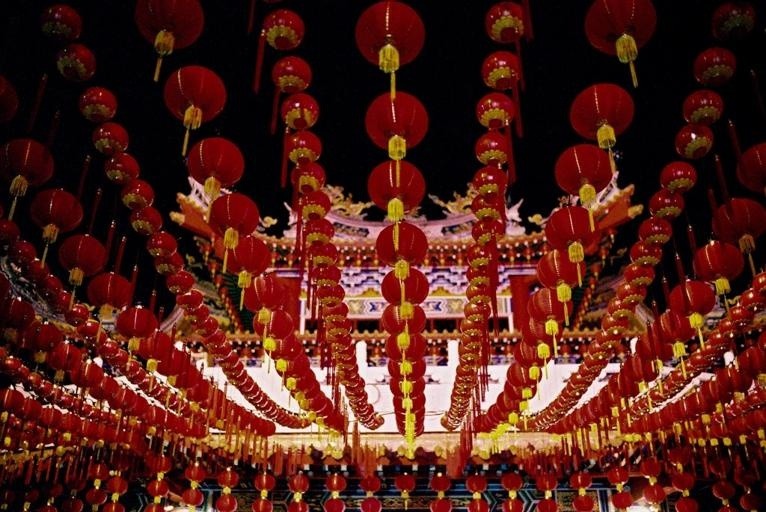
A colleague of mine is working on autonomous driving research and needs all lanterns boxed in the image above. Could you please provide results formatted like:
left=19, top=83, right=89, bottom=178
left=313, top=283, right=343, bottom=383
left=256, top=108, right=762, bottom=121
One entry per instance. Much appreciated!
left=0, top=0, right=766, bottom=512
